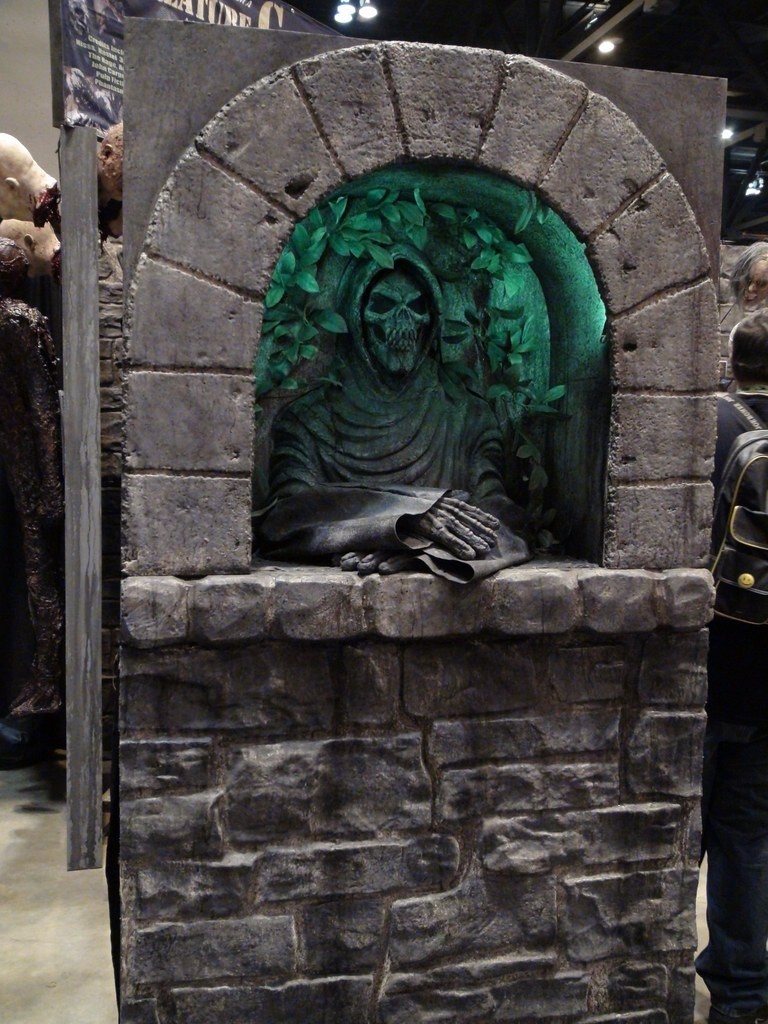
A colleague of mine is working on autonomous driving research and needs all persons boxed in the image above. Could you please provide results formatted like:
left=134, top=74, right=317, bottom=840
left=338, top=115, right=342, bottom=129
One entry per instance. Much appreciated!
left=687, top=317, right=768, bottom=1024
left=2, top=219, right=60, bottom=277
left=1, top=130, right=62, bottom=226
left=730, top=243, right=768, bottom=308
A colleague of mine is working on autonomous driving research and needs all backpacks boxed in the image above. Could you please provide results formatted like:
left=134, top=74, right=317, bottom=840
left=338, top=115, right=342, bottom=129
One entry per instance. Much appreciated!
left=712, top=392, right=768, bottom=632
